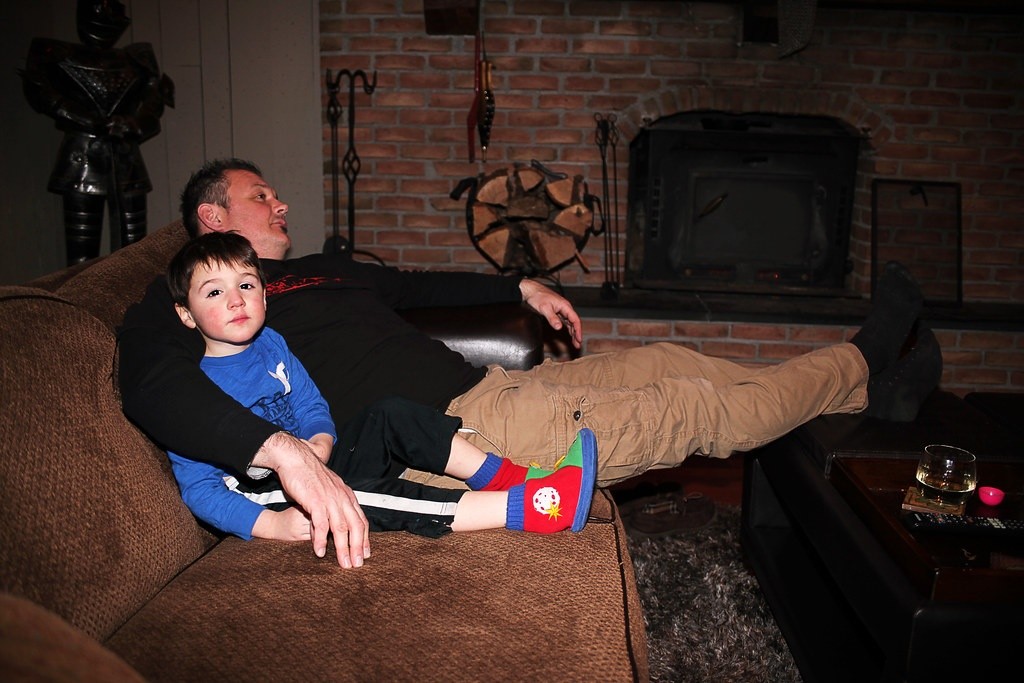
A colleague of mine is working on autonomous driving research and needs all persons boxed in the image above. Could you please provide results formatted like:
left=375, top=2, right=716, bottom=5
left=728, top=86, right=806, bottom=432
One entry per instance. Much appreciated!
left=167, top=231, right=601, bottom=543
left=20, top=1, right=176, bottom=269
left=117, top=157, right=939, bottom=568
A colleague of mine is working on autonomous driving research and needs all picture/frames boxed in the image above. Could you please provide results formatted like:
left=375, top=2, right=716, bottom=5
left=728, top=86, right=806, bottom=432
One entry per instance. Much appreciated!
left=869, top=177, right=964, bottom=305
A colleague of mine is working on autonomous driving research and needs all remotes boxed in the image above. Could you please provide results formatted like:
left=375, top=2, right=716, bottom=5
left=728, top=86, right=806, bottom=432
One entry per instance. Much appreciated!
left=902, top=511, right=1024, bottom=540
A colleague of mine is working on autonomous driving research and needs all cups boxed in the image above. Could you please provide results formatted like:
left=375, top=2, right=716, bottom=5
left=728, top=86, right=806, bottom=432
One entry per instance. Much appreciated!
left=915, top=444, right=977, bottom=505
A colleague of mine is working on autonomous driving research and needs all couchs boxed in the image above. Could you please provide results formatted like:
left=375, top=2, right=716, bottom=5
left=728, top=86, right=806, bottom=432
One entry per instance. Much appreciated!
left=0, top=220, right=659, bottom=683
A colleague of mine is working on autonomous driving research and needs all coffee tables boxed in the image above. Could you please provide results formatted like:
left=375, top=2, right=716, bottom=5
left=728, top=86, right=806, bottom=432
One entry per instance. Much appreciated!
left=741, top=387, right=1024, bottom=683
